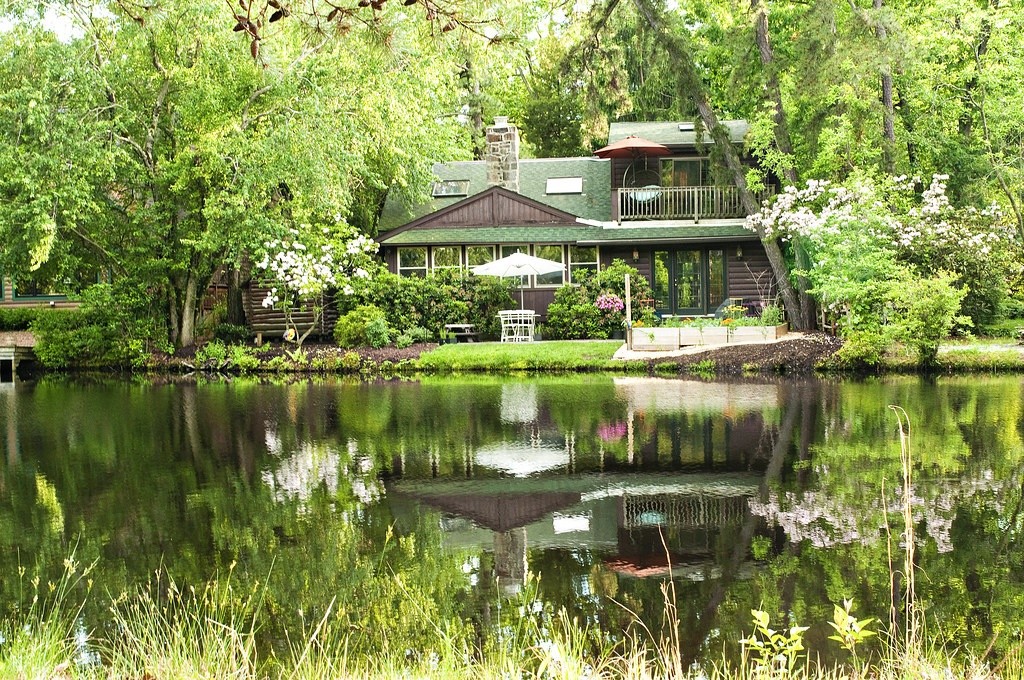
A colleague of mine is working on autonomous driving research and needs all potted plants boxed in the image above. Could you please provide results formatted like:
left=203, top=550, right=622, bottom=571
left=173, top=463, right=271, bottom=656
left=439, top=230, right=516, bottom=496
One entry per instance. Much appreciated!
left=632, top=307, right=790, bottom=351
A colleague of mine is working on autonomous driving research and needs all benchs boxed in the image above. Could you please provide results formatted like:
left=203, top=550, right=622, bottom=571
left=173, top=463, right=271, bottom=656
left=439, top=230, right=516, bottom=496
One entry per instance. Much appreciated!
left=454, top=333, right=479, bottom=336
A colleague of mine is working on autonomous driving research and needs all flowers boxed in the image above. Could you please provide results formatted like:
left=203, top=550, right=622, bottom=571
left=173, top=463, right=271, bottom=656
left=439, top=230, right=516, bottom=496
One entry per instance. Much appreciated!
left=632, top=307, right=658, bottom=328
left=595, top=294, right=626, bottom=329
left=719, top=304, right=748, bottom=326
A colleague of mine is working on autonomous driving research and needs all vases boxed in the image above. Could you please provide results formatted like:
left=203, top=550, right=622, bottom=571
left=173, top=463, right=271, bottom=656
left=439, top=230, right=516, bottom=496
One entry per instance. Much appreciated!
left=614, top=328, right=623, bottom=339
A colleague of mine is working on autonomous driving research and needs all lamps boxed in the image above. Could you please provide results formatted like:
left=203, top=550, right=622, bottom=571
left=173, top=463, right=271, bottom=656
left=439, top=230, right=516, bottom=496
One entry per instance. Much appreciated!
left=633, top=247, right=639, bottom=263
left=737, top=244, right=742, bottom=261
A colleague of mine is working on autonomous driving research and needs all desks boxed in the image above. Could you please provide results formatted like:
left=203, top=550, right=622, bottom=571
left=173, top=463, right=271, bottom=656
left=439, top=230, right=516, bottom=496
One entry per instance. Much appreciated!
left=447, top=324, right=476, bottom=343
left=496, top=314, right=541, bottom=340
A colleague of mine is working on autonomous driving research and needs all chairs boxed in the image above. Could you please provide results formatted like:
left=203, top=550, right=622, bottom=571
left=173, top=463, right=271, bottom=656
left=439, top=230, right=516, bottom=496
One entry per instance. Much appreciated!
left=499, top=310, right=535, bottom=342
left=627, top=168, right=664, bottom=204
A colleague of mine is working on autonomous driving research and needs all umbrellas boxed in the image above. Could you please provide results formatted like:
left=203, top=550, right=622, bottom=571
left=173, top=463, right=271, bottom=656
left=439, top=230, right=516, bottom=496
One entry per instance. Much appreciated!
left=470, top=444, right=580, bottom=483
left=472, top=251, right=565, bottom=341
left=592, top=134, right=674, bottom=188
